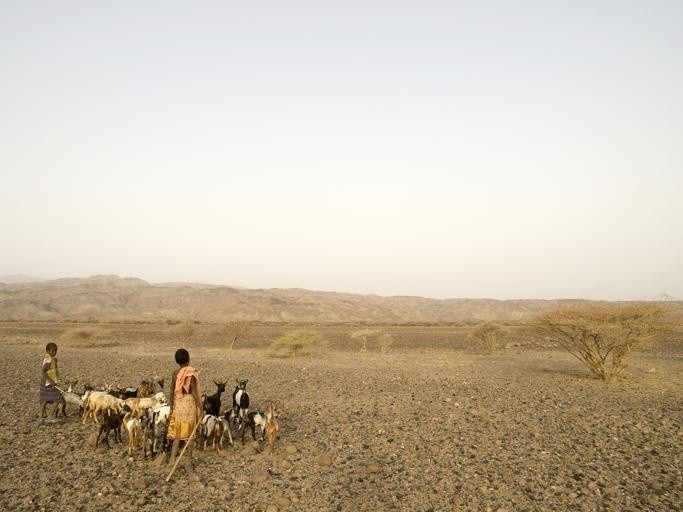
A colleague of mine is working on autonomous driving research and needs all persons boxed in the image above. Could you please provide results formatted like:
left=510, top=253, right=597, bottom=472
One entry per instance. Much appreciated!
left=165, top=348, right=203, bottom=472
left=36, top=343, right=63, bottom=421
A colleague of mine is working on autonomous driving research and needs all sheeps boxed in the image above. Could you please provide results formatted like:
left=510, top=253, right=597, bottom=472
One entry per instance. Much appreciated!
left=48, top=377, right=280, bottom=466
